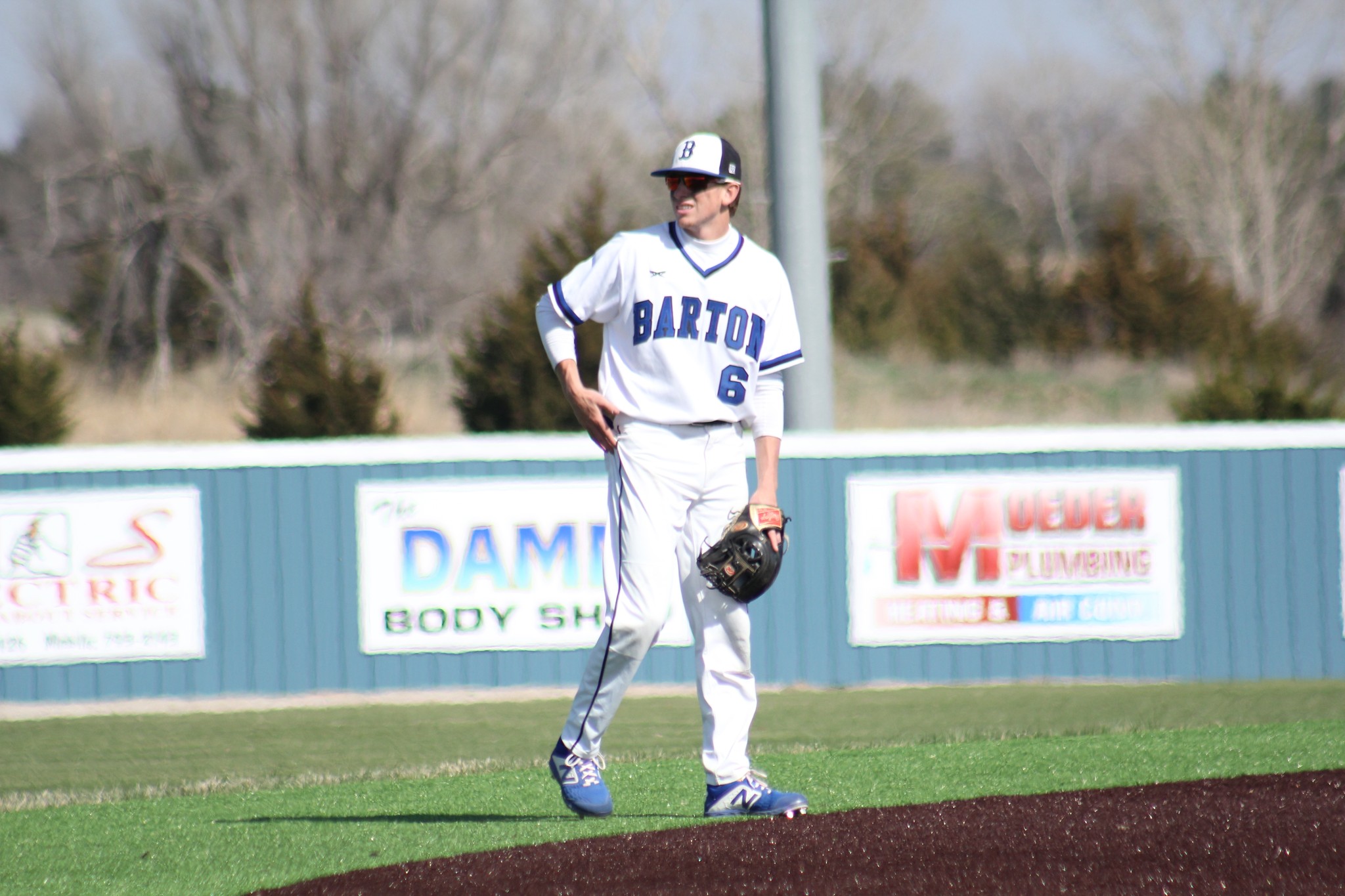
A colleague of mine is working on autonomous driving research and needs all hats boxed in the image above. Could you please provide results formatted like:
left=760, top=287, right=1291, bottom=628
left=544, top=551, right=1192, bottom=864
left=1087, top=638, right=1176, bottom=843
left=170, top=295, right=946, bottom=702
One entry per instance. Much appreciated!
left=651, top=132, right=742, bottom=182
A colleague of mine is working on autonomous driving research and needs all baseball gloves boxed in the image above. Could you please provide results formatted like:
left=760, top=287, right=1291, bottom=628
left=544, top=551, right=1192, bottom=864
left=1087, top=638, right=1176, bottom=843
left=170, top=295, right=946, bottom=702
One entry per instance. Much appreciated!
left=695, top=501, right=791, bottom=605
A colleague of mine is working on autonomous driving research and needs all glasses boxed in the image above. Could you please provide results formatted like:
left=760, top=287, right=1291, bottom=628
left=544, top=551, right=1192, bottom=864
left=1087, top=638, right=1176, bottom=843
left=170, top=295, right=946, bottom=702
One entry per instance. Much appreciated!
left=665, top=172, right=742, bottom=192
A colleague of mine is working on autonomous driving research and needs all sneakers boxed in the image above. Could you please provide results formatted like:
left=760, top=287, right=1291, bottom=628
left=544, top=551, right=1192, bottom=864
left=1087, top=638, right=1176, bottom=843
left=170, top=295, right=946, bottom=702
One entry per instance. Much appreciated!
left=704, top=769, right=808, bottom=816
left=549, top=735, right=613, bottom=817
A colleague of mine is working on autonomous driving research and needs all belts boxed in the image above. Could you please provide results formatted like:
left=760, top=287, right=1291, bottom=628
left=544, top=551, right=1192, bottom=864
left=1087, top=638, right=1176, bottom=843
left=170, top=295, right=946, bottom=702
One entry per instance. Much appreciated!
left=689, top=421, right=727, bottom=426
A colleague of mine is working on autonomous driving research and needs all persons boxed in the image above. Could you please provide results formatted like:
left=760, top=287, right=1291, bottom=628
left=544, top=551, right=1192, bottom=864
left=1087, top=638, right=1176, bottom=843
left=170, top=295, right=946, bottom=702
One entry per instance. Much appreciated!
left=534, top=132, right=807, bottom=819
